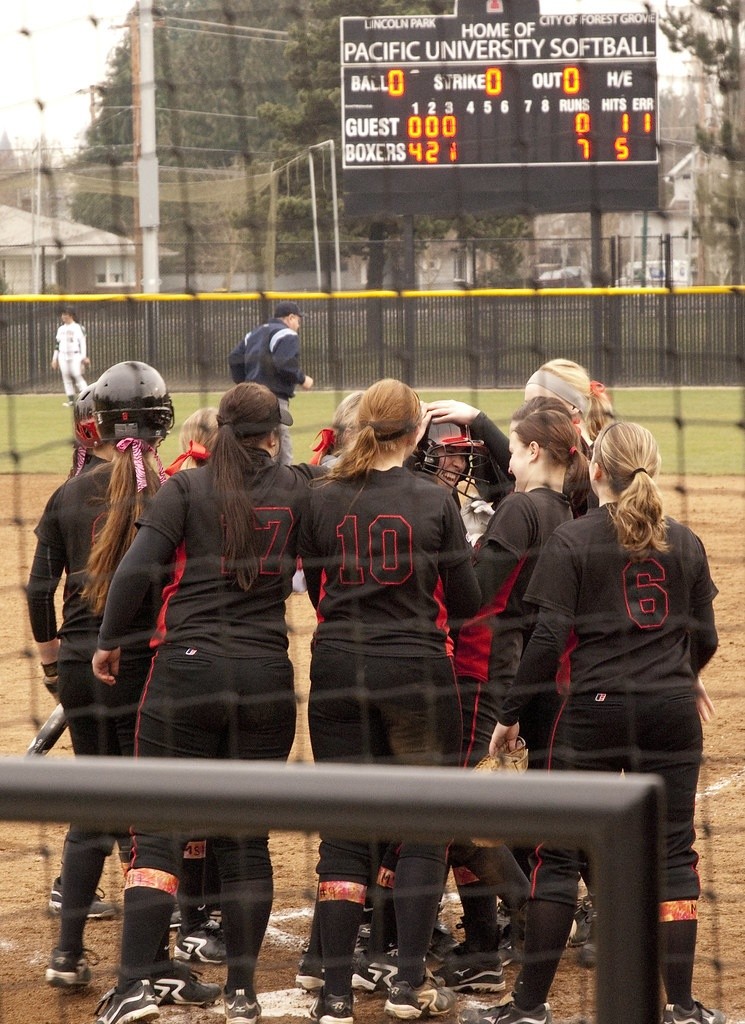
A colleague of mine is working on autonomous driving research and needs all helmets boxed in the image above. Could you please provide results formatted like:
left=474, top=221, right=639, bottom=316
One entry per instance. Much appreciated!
left=93, top=361, right=172, bottom=441
left=74, top=382, right=110, bottom=449
left=403, top=416, right=487, bottom=474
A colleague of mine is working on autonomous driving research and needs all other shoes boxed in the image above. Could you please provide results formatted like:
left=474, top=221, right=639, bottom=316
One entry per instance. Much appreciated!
left=63, top=401, right=73, bottom=406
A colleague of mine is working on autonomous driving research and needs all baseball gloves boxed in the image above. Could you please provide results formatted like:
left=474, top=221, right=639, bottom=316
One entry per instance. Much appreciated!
left=462, top=735, right=530, bottom=849
left=81, top=358, right=91, bottom=374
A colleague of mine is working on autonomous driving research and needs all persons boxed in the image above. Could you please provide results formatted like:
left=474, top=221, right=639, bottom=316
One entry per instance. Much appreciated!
left=25, top=361, right=615, bottom=1024
left=228, top=301, right=314, bottom=466
left=457, top=421, right=726, bottom=1024
left=52, top=305, right=90, bottom=408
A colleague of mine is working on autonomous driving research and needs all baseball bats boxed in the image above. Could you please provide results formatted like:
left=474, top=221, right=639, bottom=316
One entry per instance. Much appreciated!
left=25, top=701, right=67, bottom=758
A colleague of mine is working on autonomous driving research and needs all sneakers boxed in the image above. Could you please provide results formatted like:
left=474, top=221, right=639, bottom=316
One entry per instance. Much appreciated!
left=154, top=958, right=222, bottom=1007
left=169, top=907, right=223, bottom=931
left=49, top=877, right=117, bottom=918
left=46, top=947, right=100, bottom=988
left=565, top=894, right=603, bottom=945
left=173, top=920, right=229, bottom=965
left=663, top=997, right=726, bottom=1024
left=295, top=893, right=525, bottom=993
left=96, top=980, right=161, bottom=1024
left=310, top=986, right=354, bottom=1024
left=223, top=985, right=262, bottom=1024
left=458, top=991, right=553, bottom=1024
left=385, top=968, right=456, bottom=1020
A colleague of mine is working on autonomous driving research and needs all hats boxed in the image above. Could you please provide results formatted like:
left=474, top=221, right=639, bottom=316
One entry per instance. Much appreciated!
left=274, top=301, right=305, bottom=318
left=234, top=399, right=293, bottom=437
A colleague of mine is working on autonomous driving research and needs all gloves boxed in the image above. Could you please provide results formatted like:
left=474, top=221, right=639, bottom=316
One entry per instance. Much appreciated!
left=459, top=493, right=496, bottom=547
